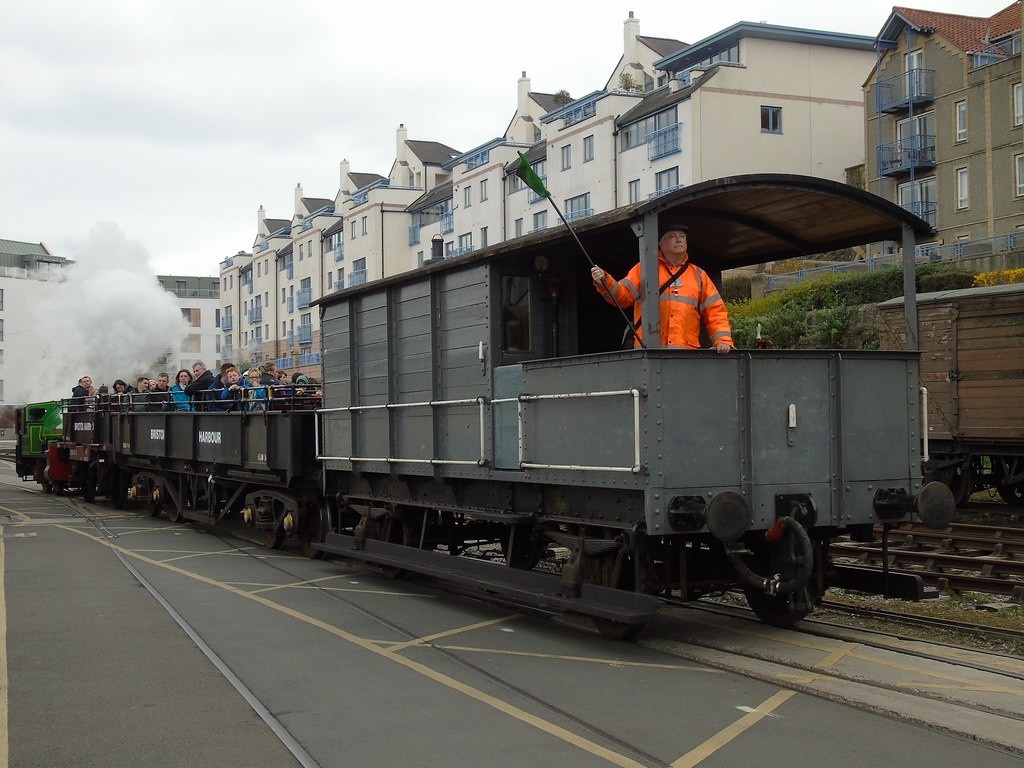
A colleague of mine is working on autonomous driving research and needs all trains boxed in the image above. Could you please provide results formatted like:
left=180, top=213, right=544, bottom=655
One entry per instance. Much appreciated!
left=0, top=171, right=1023, bottom=629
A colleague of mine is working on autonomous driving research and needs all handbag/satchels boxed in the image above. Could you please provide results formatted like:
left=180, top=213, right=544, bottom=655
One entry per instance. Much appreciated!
left=621, top=322, right=636, bottom=350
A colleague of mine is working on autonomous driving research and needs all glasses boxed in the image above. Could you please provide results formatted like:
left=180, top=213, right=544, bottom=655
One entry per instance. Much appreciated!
left=249, top=376, right=261, bottom=381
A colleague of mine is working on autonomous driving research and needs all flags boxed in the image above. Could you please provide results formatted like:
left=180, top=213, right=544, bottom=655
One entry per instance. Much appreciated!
left=516, top=155, right=551, bottom=197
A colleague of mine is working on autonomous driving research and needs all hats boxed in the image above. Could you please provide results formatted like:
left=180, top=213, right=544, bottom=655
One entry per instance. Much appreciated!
left=98, top=386, right=108, bottom=393
left=658, top=224, right=689, bottom=241
left=296, top=375, right=308, bottom=385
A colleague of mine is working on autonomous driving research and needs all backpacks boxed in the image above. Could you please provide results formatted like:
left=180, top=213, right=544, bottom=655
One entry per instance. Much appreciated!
left=249, top=384, right=269, bottom=410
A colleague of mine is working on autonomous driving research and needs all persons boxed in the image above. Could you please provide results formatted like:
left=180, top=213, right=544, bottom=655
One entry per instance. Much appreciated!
left=591, top=219, right=734, bottom=354
left=66, top=360, right=323, bottom=411
